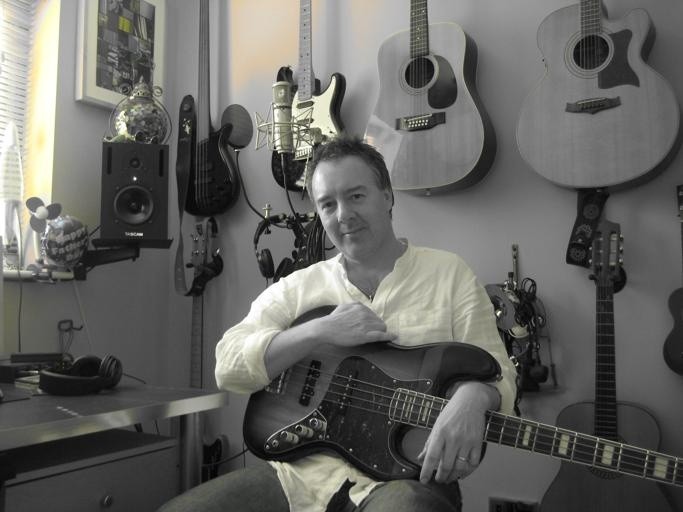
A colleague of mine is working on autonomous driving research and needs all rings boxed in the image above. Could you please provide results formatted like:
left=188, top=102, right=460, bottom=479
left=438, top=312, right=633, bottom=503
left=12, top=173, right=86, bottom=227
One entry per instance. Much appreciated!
left=457, top=456, right=468, bottom=465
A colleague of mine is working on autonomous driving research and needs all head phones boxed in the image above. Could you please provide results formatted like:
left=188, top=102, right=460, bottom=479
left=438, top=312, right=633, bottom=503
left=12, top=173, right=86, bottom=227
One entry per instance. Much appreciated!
left=38, top=352, right=123, bottom=396
left=254, top=213, right=306, bottom=283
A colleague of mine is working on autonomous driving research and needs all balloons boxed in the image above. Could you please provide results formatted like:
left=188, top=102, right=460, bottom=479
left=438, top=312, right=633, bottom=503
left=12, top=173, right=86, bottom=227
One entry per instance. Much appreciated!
left=43, top=216, right=90, bottom=267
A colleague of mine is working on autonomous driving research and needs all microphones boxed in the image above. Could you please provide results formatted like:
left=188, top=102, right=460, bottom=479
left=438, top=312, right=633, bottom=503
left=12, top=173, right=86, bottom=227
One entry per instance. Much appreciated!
left=290, top=250, right=304, bottom=268
left=270, top=79, right=295, bottom=154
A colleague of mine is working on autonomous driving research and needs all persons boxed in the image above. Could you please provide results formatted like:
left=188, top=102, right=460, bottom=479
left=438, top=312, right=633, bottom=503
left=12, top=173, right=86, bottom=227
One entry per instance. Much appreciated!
left=159, top=142, right=518, bottom=511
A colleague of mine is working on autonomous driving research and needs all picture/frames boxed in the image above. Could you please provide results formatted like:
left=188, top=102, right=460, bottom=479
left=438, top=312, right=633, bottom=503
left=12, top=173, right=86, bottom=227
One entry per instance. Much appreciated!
left=74, top=0, right=166, bottom=113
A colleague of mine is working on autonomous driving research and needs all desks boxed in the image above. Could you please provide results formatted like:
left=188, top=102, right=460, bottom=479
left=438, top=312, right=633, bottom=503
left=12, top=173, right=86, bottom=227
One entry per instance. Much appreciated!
left=0, top=382, right=231, bottom=511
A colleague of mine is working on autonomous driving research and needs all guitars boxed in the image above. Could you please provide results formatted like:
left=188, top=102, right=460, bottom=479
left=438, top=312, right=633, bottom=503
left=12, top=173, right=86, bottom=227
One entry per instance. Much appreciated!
left=175, top=0, right=240, bottom=215
left=663, top=186, right=683, bottom=375
left=270, top=0, right=347, bottom=193
left=541, top=221, right=677, bottom=508
left=243, top=305, right=683, bottom=487
left=364, top=0, right=496, bottom=195
left=515, top=0, right=681, bottom=192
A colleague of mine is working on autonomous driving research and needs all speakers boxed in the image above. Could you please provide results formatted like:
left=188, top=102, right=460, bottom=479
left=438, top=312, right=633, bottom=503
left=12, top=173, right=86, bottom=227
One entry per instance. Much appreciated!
left=99, top=141, right=170, bottom=240
